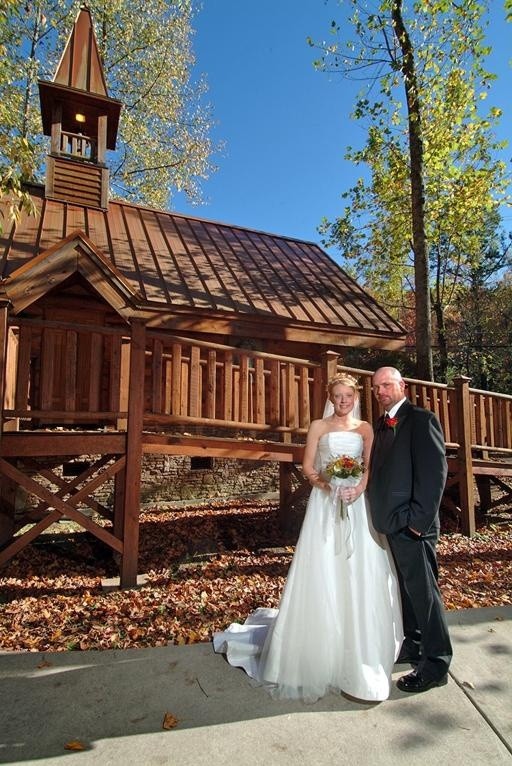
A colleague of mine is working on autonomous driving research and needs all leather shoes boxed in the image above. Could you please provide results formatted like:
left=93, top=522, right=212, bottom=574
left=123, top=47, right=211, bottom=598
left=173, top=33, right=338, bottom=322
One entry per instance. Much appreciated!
left=396, top=638, right=448, bottom=692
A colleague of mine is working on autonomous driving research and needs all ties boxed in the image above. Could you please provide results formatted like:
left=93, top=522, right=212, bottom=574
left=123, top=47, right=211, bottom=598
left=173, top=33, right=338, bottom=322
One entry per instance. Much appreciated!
left=381, top=414, right=391, bottom=428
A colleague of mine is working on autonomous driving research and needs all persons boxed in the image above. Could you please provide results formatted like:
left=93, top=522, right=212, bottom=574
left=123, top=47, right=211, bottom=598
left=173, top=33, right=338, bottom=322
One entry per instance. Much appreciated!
left=365, top=367, right=453, bottom=703
left=302, top=366, right=406, bottom=703
